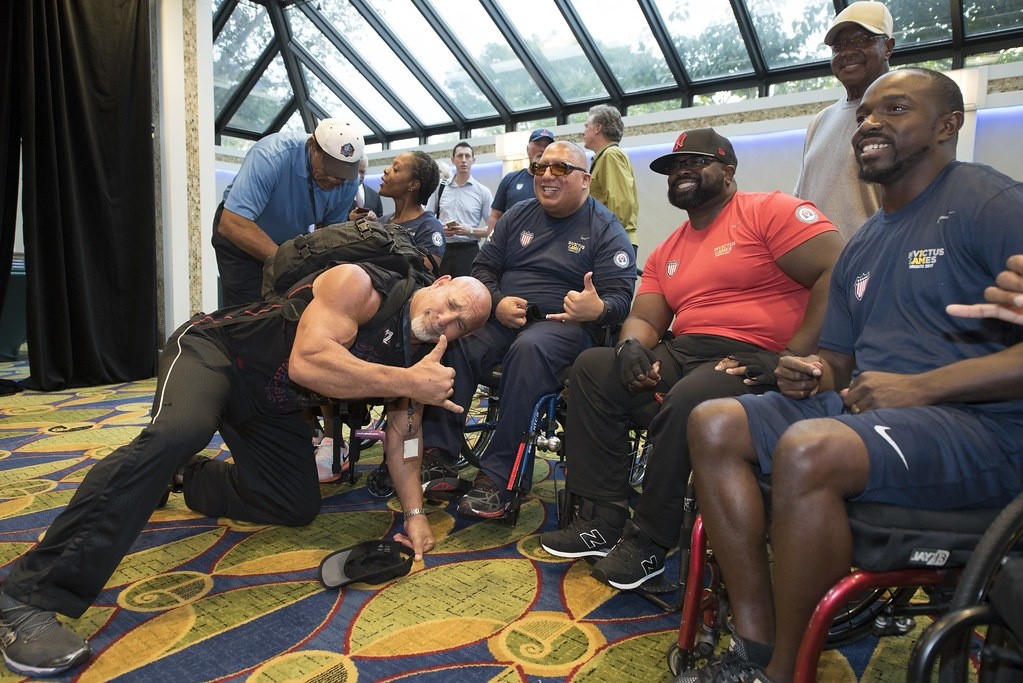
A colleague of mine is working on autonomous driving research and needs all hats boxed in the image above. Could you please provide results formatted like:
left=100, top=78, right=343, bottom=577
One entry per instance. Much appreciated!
left=529, top=129, right=556, bottom=143
left=824, top=1, right=894, bottom=43
left=315, top=118, right=365, bottom=181
left=649, top=128, right=739, bottom=176
left=317, top=539, right=415, bottom=591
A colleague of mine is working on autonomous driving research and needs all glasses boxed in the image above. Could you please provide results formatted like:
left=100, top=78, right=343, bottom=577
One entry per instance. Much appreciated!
left=830, top=34, right=888, bottom=54
left=665, top=156, right=730, bottom=175
left=529, top=161, right=587, bottom=176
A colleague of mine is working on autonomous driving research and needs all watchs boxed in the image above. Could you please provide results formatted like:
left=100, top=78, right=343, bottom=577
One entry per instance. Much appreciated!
left=467, top=225, right=474, bottom=237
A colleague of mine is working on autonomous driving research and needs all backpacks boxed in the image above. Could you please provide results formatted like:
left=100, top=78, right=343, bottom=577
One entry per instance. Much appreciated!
left=260, top=215, right=432, bottom=408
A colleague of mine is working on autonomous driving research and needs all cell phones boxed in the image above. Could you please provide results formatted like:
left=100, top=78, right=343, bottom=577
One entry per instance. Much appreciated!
left=356, top=207, right=370, bottom=214
left=446, top=220, right=457, bottom=231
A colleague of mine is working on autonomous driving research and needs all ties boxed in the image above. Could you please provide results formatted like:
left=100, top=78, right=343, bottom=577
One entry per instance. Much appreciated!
left=356, top=187, right=364, bottom=209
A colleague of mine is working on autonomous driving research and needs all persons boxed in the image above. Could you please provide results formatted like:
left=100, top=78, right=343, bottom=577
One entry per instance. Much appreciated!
left=0, top=261, right=492, bottom=678
left=794, top=1, right=896, bottom=240
left=582, top=104, right=638, bottom=261
left=664, top=66, right=1023, bottom=683
left=488, top=128, right=557, bottom=238
left=212, top=120, right=364, bottom=310
left=417, top=141, right=636, bottom=519
left=377, top=150, right=446, bottom=273
left=537, top=129, right=847, bottom=591
left=354, top=154, right=384, bottom=218
left=423, top=143, right=494, bottom=280
left=946, top=254, right=1023, bottom=327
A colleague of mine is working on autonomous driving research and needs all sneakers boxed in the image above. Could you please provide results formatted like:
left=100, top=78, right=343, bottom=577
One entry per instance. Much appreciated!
left=671, top=630, right=776, bottom=683
left=0, top=586, right=93, bottom=678
left=311, top=429, right=324, bottom=454
left=419, top=448, right=460, bottom=495
left=456, top=472, right=514, bottom=520
left=538, top=516, right=624, bottom=558
left=314, top=437, right=350, bottom=483
left=154, top=471, right=185, bottom=510
left=590, top=519, right=666, bottom=590
